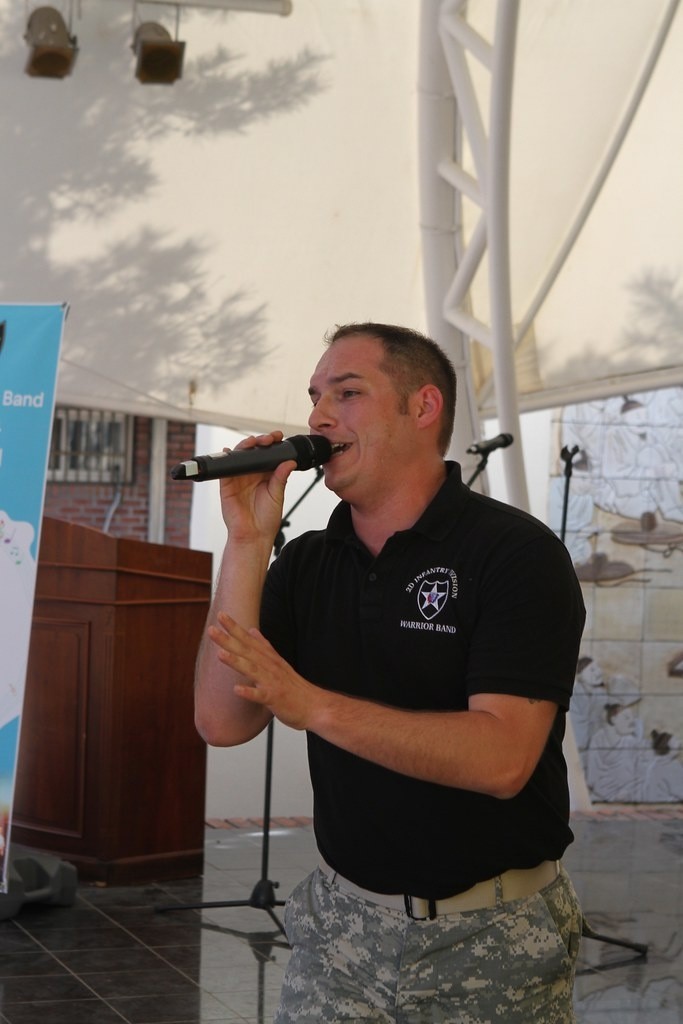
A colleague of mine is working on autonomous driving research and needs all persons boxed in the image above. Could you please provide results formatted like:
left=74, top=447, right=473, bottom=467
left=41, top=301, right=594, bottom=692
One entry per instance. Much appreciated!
left=188, top=323, right=590, bottom=1024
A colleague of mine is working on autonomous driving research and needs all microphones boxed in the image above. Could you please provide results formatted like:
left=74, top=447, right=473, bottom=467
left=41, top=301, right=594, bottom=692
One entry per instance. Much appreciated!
left=466, top=433, right=513, bottom=455
left=170, top=434, right=331, bottom=483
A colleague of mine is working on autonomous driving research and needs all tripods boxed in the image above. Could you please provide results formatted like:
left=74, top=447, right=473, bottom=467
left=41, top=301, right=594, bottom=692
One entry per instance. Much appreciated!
left=155, top=496, right=313, bottom=943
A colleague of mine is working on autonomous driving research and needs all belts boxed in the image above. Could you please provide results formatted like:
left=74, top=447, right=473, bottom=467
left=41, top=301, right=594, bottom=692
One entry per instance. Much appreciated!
left=318, top=856, right=560, bottom=919
